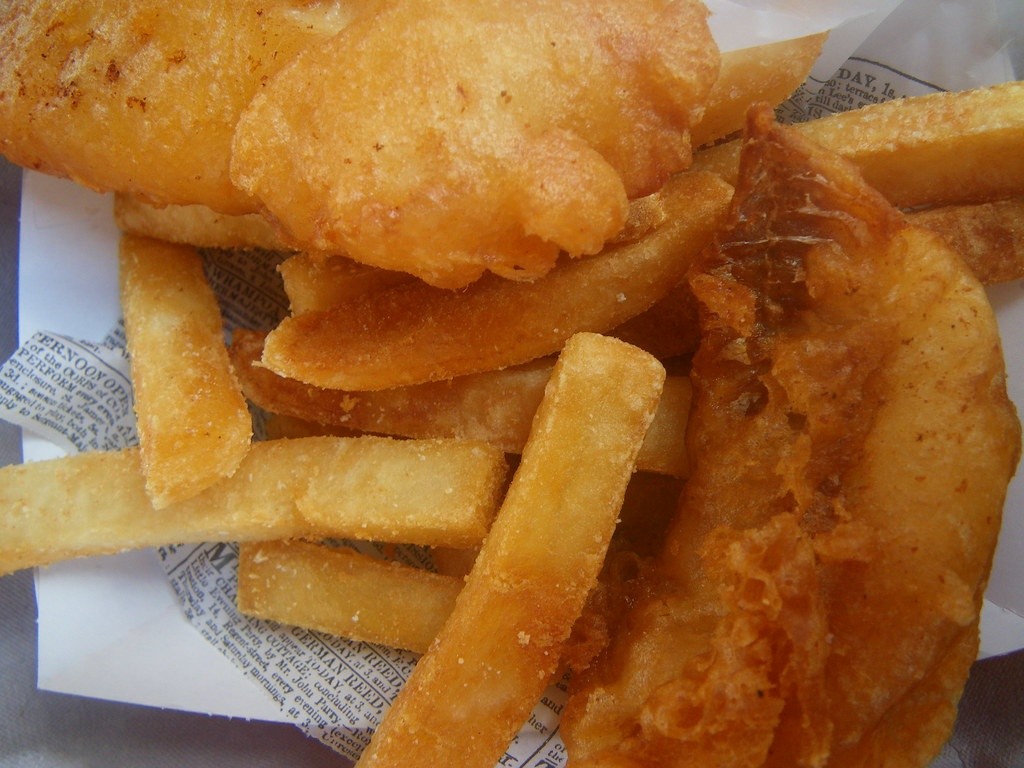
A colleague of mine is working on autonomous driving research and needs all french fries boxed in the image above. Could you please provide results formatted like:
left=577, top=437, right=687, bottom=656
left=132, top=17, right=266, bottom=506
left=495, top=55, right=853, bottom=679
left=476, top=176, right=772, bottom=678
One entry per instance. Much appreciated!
left=0, top=30, right=1024, bottom=768
